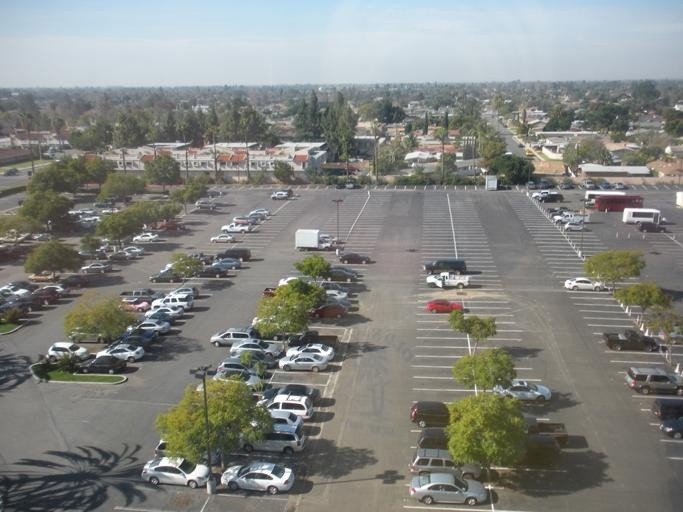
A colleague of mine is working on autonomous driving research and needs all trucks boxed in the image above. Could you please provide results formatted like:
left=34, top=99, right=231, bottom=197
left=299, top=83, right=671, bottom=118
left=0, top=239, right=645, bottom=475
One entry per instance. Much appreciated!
left=47, top=146, right=59, bottom=159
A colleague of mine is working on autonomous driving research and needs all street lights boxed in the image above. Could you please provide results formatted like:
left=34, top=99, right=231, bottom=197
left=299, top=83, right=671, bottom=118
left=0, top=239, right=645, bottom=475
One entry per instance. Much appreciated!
left=577, top=197, right=591, bottom=257
left=372, top=135, right=381, bottom=185
left=637, top=110, right=648, bottom=135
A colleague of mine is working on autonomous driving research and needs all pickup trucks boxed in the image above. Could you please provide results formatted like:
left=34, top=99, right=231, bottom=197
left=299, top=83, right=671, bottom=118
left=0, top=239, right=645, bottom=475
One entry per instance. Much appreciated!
left=604, top=330, right=658, bottom=352
left=425, top=272, right=470, bottom=289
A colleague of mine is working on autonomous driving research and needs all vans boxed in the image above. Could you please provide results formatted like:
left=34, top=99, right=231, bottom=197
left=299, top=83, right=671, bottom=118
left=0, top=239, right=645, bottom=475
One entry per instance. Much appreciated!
left=421, top=258, right=468, bottom=275
left=652, top=397, right=683, bottom=419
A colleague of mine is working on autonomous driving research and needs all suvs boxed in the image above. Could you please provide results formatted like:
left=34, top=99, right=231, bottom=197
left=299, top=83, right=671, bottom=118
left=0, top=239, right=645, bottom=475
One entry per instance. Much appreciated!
left=504, top=152, right=512, bottom=159
left=624, top=366, right=683, bottom=395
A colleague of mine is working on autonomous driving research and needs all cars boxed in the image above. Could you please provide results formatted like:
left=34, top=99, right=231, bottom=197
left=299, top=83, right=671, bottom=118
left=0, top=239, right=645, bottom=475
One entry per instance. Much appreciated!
left=142, top=373, right=323, bottom=494
left=658, top=326, right=683, bottom=344
left=565, top=277, right=604, bottom=292
left=660, top=416, right=683, bottom=439
left=425, top=298, right=463, bottom=313
left=525, top=150, right=533, bottom=156
left=408, top=377, right=590, bottom=506
left=518, top=144, right=523, bottom=148
left=526, top=180, right=667, bottom=233
